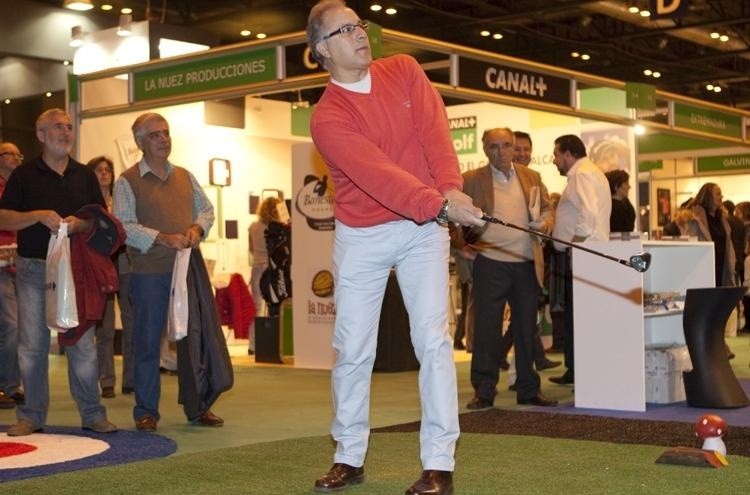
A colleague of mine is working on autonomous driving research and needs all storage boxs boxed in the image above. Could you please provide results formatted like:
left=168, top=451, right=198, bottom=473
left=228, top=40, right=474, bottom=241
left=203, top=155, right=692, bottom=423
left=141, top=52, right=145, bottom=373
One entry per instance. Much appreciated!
left=645, top=342, right=693, bottom=405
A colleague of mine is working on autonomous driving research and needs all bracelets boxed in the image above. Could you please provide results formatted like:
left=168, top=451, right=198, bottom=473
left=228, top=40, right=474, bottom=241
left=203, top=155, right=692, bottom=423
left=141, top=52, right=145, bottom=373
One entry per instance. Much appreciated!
left=436, top=197, right=451, bottom=226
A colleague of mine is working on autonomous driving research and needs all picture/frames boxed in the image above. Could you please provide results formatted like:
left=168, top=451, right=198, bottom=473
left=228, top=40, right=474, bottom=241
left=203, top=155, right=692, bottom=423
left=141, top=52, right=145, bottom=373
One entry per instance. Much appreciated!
left=657, top=188, right=671, bottom=226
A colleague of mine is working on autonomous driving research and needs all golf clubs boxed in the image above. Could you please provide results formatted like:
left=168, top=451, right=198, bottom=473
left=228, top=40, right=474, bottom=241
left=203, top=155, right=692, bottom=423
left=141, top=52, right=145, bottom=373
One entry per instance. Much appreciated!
left=481, top=215, right=651, bottom=272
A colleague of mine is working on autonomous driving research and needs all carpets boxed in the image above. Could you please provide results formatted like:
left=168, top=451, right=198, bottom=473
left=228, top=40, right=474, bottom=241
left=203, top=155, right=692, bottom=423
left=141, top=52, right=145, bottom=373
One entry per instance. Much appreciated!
left=0, top=405, right=749, bottom=495
left=0, top=424, right=177, bottom=482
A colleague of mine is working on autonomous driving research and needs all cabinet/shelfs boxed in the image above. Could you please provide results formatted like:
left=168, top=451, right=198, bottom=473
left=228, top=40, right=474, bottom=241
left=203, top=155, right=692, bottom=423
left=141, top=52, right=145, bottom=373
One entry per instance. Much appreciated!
left=572, top=240, right=716, bottom=412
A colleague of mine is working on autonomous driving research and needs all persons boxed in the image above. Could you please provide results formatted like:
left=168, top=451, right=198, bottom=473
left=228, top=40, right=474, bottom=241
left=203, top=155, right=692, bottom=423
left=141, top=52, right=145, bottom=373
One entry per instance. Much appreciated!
left=247, top=196, right=283, bottom=353
left=308, top=1, right=488, bottom=495
left=449, top=126, right=612, bottom=409
left=0, top=108, right=128, bottom=436
left=665, top=182, right=750, bottom=359
left=113, top=113, right=227, bottom=431
left=85, top=154, right=135, bottom=399
left=1, top=141, right=27, bottom=409
left=605, top=170, right=637, bottom=233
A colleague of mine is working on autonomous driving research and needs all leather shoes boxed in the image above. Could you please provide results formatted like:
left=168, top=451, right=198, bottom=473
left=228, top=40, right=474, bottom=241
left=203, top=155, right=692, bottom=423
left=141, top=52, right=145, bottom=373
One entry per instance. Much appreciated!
left=135, top=414, right=157, bottom=432
left=466, top=395, right=493, bottom=410
left=195, top=411, right=224, bottom=427
left=314, top=462, right=364, bottom=492
left=405, top=469, right=454, bottom=495
left=517, top=394, right=558, bottom=407
left=536, top=360, right=573, bottom=385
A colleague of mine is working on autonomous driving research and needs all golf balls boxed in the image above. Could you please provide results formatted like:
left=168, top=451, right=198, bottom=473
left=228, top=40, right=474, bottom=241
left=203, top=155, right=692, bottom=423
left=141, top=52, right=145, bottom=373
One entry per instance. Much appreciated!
left=695, top=415, right=725, bottom=438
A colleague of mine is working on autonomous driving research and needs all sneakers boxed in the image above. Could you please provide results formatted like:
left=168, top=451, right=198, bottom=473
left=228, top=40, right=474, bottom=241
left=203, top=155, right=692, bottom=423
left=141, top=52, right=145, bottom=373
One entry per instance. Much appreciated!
left=102, top=386, right=116, bottom=398
left=7, top=419, right=37, bottom=436
left=83, top=419, right=117, bottom=433
left=0, top=388, right=24, bottom=409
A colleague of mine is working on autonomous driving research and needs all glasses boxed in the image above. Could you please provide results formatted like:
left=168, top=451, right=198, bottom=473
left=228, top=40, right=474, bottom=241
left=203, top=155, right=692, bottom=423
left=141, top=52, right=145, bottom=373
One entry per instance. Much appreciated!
left=322, top=20, right=370, bottom=41
left=0, top=152, right=24, bottom=160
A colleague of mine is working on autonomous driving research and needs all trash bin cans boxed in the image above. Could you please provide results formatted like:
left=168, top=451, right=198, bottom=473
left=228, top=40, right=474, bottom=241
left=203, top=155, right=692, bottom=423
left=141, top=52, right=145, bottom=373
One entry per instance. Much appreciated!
left=365, top=269, right=421, bottom=373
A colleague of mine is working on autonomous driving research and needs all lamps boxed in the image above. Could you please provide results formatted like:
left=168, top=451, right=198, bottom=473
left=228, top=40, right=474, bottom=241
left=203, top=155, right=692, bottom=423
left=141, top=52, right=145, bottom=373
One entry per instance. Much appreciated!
left=116, top=14, right=133, bottom=37
left=71, top=25, right=83, bottom=42
